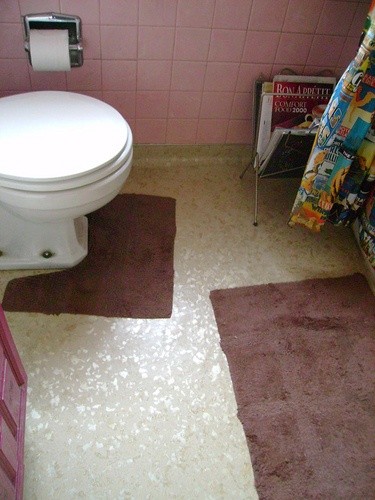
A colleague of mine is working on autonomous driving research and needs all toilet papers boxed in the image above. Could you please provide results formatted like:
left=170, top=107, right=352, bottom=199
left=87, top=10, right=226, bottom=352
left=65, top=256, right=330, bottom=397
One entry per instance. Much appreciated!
left=29, top=29, right=71, bottom=72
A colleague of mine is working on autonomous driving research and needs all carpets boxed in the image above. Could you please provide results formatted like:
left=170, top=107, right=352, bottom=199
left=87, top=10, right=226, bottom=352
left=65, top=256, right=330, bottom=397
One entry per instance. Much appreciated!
left=209, top=273, right=374, bottom=500
left=2, top=193, right=177, bottom=319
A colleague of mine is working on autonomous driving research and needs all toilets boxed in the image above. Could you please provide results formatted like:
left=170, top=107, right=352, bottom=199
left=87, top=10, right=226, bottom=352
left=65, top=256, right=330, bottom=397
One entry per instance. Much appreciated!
left=0, top=90, right=135, bottom=270
